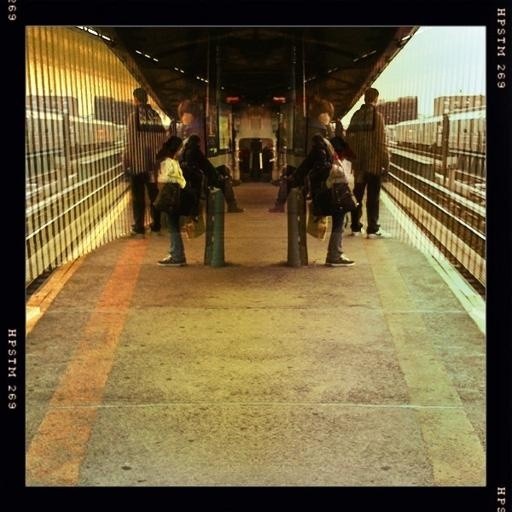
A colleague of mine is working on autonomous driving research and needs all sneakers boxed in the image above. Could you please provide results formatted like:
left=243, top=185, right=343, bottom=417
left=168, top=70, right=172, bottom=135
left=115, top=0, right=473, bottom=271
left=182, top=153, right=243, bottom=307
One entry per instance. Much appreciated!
left=351, top=228, right=392, bottom=239
left=270, top=177, right=286, bottom=186
left=121, top=229, right=159, bottom=240
left=227, top=207, right=245, bottom=213
left=156, top=257, right=185, bottom=267
left=268, top=205, right=284, bottom=212
left=224, top=177, right=243, bottom=188
left=326, top=259, right=356, bottom=267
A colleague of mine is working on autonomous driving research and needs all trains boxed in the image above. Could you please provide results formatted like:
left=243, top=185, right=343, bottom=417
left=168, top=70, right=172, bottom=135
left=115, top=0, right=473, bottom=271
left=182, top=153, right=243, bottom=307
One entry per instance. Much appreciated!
left=25, top=106, right=127, bottom=157
left=384, top=111, right=489, bottom=158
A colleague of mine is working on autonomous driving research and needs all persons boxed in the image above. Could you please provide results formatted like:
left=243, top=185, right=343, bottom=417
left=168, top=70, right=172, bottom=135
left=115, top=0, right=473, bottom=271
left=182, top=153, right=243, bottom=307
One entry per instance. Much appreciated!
left=122, top=88, right=246, bottom=267
left=268, top=88, right=391, bottom=266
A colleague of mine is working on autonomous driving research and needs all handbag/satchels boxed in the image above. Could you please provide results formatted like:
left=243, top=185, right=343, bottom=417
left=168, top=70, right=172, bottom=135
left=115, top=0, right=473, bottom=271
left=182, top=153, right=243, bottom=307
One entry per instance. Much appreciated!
left=306, top=205, right=328, bottom=239
left=188, top=217, right=207, bottom=239
left=152, top=185, right=192, bottom=215
left=312, top=183, right=359, bottom=216
